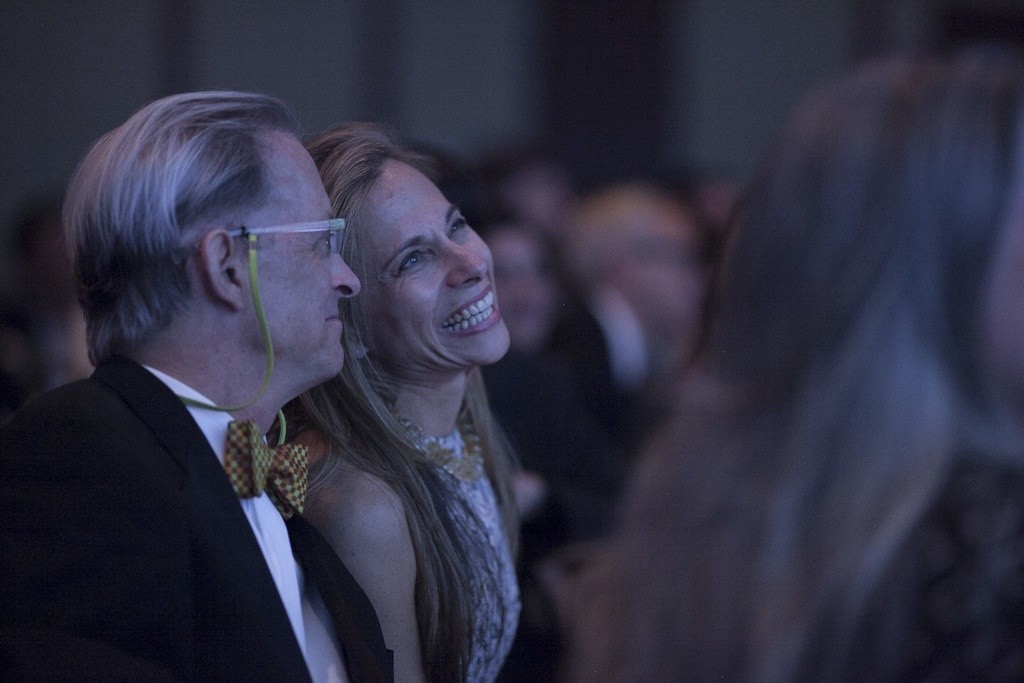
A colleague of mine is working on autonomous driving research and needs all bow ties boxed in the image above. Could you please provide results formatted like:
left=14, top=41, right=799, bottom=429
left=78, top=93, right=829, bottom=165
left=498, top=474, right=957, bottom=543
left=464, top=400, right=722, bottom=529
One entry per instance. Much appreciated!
left=225, top=417, right=308, bottom=518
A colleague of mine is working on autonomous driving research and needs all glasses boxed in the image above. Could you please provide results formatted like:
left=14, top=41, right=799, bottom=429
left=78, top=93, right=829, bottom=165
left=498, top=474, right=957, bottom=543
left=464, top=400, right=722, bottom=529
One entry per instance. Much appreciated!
left=192, top=210, right=347, bottom=254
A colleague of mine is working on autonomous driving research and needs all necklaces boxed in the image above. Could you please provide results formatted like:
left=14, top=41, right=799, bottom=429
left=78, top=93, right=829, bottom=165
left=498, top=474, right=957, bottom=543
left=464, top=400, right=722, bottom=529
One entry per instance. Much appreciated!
left=393, top=400, right=485, bottom=483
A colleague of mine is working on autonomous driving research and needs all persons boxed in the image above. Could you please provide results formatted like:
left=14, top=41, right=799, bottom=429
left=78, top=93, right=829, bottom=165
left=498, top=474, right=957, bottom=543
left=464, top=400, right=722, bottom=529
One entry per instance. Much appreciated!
left=265, top=118, right=524, bottom=683
left=0, top=90, right=396, bottom=683
left=431, top=52, right=1024, bottom=683
